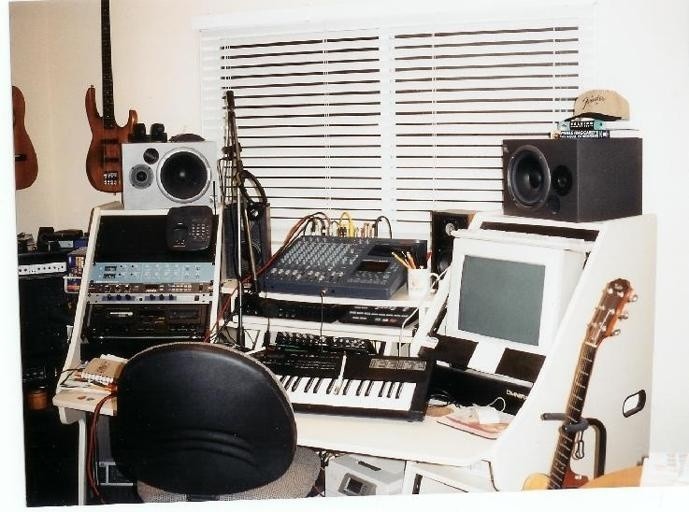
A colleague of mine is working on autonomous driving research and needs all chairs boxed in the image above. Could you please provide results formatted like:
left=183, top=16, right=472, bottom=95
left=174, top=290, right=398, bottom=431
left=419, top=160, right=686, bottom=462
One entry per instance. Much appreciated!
left=112, top=341, right=323, bottom=503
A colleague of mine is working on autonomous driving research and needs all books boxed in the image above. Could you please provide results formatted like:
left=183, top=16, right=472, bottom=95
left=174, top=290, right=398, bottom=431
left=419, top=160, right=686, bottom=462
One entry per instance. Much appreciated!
left=551, top=129, right=642, bottom=139
left=558, top=115, right=638, bottom=131
left=56, top=349, right=131, bottom=390
left=435, top=410, right=518, bottom=440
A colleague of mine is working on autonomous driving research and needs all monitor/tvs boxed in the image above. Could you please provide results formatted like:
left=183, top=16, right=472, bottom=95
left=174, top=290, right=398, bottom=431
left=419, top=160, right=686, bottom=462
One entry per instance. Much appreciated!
left=443, top=237, right=587, bottom=375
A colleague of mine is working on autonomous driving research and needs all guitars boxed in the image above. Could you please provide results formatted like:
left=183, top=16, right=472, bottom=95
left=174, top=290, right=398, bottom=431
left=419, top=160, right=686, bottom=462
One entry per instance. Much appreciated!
left=523, top=279, right=640, bottom=489
left=85, top=0, right=137, bottom=193
left=12, top=85, right=37, bottom=189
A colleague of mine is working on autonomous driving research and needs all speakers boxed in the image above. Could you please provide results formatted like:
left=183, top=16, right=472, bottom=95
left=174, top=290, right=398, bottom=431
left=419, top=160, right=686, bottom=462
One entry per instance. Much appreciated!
left=430, top=208, right=478, bottom=289
left=120, top=141, right=221, bottom=211
left=230, top=200, right=272, bottom=276
left=502, top=136, right=643, bottom=223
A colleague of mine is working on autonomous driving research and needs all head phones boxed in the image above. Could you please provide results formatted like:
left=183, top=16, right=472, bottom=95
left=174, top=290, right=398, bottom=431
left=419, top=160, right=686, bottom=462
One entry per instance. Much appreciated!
left=235, top=170, right=268, bottom=222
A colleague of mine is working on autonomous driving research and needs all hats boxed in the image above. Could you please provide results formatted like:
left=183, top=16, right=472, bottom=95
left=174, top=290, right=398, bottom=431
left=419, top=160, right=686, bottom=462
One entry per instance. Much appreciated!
left=564, top=90, right=630, bottom=122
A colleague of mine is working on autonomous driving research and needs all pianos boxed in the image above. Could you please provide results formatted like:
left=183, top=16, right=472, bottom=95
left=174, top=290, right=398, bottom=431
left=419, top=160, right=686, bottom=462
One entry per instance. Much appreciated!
left=258, top=350, right=434, bottom=420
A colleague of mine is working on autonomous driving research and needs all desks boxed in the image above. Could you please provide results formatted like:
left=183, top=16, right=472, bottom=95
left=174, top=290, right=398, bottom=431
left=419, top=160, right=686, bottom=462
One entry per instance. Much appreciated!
left=50, top=210, right=653, bottom=505
